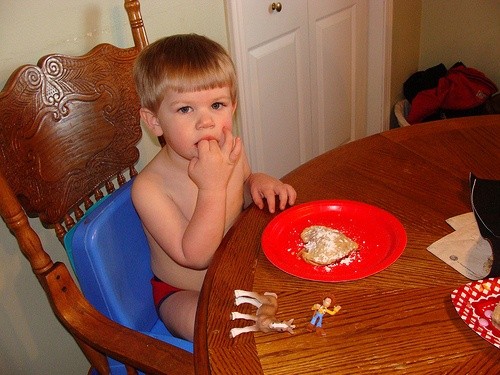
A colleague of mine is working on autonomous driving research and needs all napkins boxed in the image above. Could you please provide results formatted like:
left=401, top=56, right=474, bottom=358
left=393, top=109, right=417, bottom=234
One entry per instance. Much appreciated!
left=426, top=211, right=493, bottom=281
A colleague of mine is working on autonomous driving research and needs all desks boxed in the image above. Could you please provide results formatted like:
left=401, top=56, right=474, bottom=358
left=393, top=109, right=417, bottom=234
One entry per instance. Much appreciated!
left=192, top=114, right=500, bottom=375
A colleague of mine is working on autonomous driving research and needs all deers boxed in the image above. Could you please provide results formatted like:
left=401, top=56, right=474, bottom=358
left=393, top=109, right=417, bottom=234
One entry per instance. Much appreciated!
left=228, top=290, right=296, bottom=338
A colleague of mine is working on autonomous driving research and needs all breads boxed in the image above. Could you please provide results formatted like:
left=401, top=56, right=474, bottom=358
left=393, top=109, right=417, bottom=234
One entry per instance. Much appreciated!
left=491, top=300, right=500, bottom=325
left=301, top=225, right=359, bottom=266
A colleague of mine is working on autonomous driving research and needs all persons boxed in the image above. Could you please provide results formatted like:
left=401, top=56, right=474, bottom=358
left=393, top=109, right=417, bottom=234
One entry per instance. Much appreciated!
left=131, top=33, right=297, bottom=343
left=306, top=292, right=341, bottom=335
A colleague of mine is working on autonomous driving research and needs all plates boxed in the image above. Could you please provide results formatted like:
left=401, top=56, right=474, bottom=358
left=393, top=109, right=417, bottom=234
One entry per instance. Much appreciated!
left=262, top=199, right=407, bottom=282
left=451, top=278, right=500, bottom=348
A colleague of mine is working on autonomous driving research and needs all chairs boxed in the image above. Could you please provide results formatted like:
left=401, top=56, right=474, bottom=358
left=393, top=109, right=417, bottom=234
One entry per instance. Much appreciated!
left=0, top=1, right=197, bottom=375
